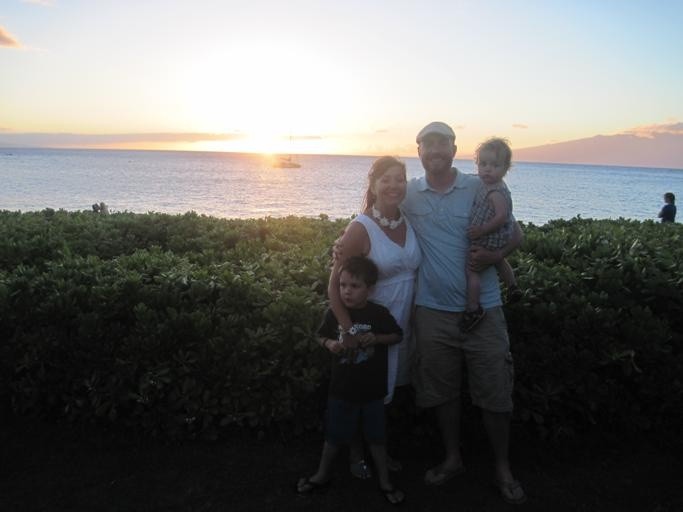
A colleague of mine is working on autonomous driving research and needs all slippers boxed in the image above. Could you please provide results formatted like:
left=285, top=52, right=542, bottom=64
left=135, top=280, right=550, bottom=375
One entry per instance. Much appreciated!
left=349, top=454, right=403, bottom=481
left=423, top=462, right=469, bottom=486
left=494, top=468, right=529, bottom=506
left=378, top=479, right=405, bottom=504
left=295, top=475, right=332, bottom=493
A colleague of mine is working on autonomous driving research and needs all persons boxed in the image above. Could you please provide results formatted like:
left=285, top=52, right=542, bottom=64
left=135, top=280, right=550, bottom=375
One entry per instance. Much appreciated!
left=98, top=202, right=109, bottom=215
left=456, top=139, right=522, bottom=334
left=326, top=155, right=422, bottom=483
left=329, top=121, right=528, bottom=507
left=657, top=192, right=676, bottom=224
left=292, top=253, right=407, bottom=506
left=92, top=204, right=99, bottom=213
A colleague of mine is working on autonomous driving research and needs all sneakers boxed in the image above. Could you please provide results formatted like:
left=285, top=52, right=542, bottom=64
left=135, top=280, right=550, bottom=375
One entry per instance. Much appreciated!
left=504, top=284, right=522, bottom=305
left=458, top=305, right=485, bottom=333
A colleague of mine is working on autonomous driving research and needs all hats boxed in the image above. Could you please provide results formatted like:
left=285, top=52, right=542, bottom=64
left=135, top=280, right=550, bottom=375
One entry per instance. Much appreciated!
left=414, top=121, right=456, bottom=144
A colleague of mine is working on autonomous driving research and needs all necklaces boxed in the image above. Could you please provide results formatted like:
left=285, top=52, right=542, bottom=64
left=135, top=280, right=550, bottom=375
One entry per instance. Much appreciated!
left=370, top=201, right=404, bottom=231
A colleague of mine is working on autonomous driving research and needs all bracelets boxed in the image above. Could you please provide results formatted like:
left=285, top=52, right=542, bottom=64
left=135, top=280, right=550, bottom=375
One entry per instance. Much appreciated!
left=321, top=337, right=330, bottom=352
left=340, top=323, right=357, bottom=337
